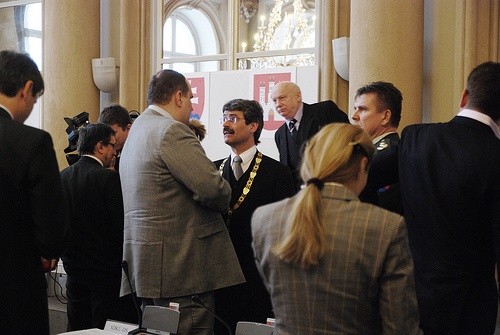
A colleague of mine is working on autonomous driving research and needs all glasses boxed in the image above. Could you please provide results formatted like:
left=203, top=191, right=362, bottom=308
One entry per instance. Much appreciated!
left=220, top=116, right=245, bottom=124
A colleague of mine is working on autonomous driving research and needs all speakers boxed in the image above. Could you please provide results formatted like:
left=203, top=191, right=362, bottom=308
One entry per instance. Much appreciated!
left=92, top=57, right=116, bottom=92
left=332, top=36, right=351, bottom=81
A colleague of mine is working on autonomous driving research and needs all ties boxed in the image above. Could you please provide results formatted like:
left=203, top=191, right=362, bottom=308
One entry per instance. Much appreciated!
left=289, top=119, right=297, bottom=135
left=232, top=156, right=243, bottom=180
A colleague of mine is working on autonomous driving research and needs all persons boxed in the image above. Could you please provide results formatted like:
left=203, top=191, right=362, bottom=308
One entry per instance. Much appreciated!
left=350, top=81, right=403, bottom=202
left=118, top=70, right=246, bottom=335
left=78, top=105, right=206, bottom=176
left=60, top=123, right=123, bottom=332
left=272, top=81, right=349, bottom=188
left=211, top=99, right=300, bottom=335
left=365, top=61, right=500, bottom=335
left=0, top=50, right=63, bottom=335
left=250, top=122, right=419, bottom=335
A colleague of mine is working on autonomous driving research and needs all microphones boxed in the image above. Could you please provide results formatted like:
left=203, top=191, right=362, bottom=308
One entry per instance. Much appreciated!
left=121, top=260, right=146, bottom=334
left=192, top=295, right=232, bottom=335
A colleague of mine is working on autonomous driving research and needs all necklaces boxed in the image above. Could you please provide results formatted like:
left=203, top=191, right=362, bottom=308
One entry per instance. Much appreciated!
left=218, top=151, right=262, bottom=215
